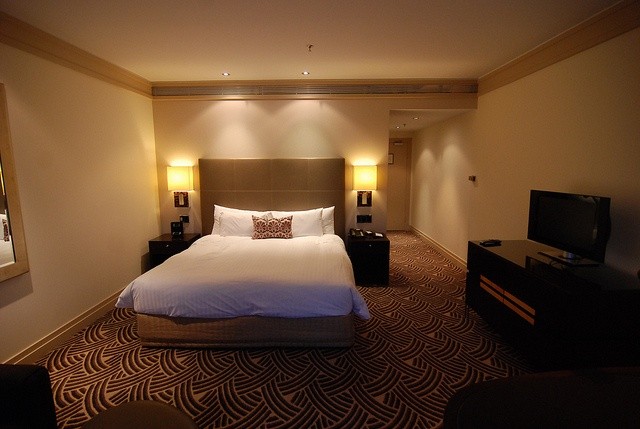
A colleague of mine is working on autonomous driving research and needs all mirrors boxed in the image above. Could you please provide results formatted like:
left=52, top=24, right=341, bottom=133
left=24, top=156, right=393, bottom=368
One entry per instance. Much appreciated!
left=0, top=83, right=29, bottom=282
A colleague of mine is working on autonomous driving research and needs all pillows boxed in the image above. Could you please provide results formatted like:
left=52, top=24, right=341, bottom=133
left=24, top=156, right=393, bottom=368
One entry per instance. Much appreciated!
left=212, top=205, right=268, bottom=238
left=286, top=210, right=324, bottom=238
left=253, top=216, right=292, bottom=239
left=220, top=213, right=253, bottom=237
left=274, top=206, right=335, bottom=234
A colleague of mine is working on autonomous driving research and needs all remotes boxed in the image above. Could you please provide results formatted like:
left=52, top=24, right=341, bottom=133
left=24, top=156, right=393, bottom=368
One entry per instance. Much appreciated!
left=479, top=239, right=502, bottom=245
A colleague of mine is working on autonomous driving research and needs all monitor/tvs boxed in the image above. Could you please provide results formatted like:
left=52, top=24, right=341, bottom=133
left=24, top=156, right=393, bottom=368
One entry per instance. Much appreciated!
left=527, top=189, right=611, bottom=267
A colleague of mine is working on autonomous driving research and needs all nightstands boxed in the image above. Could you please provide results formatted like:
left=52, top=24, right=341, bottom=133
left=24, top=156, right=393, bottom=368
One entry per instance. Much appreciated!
left=345, top=230, right=390, bottom=287
left=149, top=233, right=199, bottom=265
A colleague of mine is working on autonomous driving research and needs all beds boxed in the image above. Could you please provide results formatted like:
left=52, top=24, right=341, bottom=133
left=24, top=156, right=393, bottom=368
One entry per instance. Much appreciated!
left=115, top=205, right=369, bottom=348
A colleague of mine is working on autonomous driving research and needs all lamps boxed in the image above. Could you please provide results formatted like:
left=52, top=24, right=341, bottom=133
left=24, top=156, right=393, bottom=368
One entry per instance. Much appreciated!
left=164, top=166, right=196, bottom=207
left=351, top=163, right=377, bottom=206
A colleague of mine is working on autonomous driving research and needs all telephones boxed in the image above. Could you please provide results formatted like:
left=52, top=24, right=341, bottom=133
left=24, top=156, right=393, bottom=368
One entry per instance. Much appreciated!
left=348, top=228, right=366, bottom=238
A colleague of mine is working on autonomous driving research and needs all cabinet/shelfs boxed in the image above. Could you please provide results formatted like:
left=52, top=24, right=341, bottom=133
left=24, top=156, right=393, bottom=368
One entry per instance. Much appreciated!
left=466, top=236, right=606, bottom=370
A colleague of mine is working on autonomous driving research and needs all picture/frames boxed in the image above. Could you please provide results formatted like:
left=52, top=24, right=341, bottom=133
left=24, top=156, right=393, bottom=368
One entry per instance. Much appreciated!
left=388, top=152, right=394, bottom=165
